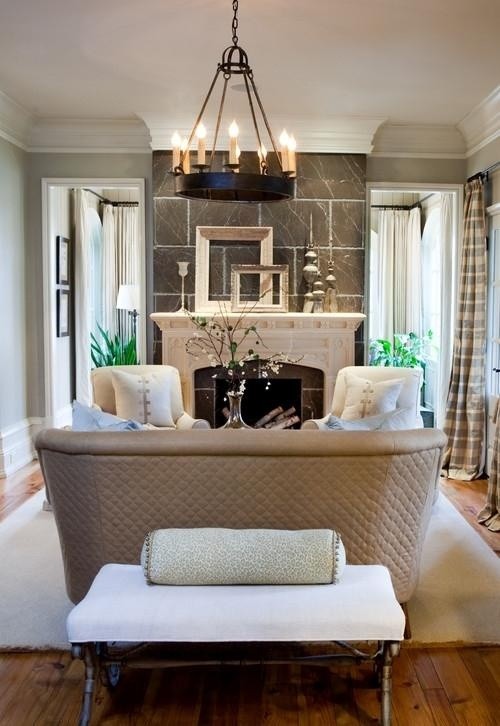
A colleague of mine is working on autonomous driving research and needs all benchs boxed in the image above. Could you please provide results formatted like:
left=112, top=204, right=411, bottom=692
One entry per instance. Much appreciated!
left=66, top=563, right=407, bottom=726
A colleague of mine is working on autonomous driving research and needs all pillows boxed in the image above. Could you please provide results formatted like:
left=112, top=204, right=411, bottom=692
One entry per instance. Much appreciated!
left=110, top=369, right=176, bottom=429
left=139, top=528, right=345, bottom=586
left=340, top=374, right=406, bottom=420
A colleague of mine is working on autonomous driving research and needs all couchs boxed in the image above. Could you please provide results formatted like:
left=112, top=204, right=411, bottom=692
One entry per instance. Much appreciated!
left=36, top=426, right=448, bottom=607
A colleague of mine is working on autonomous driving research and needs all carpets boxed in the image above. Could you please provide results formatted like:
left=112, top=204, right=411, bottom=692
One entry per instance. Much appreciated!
left=0, top=483, right=500, bottom=653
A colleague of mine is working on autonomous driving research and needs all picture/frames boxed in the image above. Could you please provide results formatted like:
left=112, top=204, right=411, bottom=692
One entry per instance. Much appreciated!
left=56, top=235, right=69, bottom=285
left=56, top=288, right=70, bottom=337
left=194, top=226, right=272, bottom=313
left=230, top=262, right=290, bottom=313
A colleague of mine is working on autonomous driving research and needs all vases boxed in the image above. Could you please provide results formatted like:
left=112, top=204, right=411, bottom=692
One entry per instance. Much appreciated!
left=217, top=393, right=253, bottom=429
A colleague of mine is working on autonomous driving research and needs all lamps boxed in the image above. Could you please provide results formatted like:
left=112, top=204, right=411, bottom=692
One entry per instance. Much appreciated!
left=168, top=0, right=298, bottom=204
left=116, top=284, right=139, bottom=335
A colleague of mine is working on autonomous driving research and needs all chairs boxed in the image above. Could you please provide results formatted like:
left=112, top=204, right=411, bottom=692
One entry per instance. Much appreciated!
left=90, top=364, right=213, bottom=429
left=299, top=364, right=424, bottom=432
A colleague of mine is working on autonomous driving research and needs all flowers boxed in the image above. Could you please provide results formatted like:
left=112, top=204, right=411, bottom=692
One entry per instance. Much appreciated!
left=181, top=286, right=305, bottom=394
